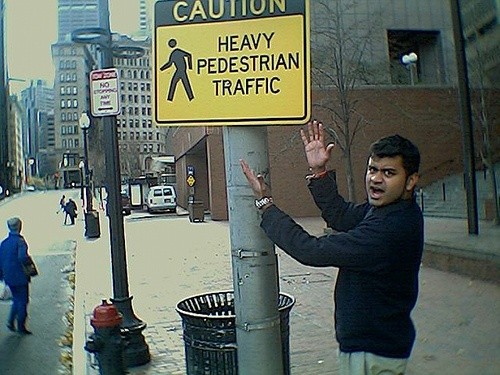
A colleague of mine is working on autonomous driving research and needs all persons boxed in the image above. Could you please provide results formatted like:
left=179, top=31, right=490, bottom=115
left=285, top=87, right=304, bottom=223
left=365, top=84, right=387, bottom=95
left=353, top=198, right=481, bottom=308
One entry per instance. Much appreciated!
left=0, top=217, right=37, bottom=335
left=61, top=194, right=78, bottom=225
left=240, top=120, right=425, bottom=375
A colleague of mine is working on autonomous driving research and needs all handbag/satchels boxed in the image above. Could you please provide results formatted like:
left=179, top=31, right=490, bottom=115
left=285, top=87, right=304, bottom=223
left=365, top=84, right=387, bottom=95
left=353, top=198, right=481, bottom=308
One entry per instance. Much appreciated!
left=0, top=286, right=14, bottom=300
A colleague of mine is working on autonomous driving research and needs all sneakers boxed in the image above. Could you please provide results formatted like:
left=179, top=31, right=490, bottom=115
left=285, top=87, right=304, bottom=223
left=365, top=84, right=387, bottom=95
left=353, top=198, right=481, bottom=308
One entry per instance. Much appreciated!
left=18, top=326, right=32, bottom=335
left=5, top=322, right=16, bottom=332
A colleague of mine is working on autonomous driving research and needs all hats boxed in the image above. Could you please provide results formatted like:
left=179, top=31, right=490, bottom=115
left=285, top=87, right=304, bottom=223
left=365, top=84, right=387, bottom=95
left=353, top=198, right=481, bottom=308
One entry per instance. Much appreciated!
left=7, top=218, right=21, bottom=230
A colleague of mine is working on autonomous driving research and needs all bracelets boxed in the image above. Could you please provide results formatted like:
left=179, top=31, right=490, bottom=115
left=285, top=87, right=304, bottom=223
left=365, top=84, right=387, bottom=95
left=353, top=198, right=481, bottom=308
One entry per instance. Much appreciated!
left=304, top=168, right=329, bottom=179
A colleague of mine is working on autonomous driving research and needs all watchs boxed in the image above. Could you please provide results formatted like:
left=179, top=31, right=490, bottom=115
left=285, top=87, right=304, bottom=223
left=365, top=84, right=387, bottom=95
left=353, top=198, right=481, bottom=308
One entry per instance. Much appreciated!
left=255, top=197, right=273, bottom=209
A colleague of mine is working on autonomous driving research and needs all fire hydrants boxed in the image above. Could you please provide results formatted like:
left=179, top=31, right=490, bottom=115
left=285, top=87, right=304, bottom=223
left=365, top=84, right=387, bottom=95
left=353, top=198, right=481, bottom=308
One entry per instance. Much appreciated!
left=85, top=299, right=130, bottom=375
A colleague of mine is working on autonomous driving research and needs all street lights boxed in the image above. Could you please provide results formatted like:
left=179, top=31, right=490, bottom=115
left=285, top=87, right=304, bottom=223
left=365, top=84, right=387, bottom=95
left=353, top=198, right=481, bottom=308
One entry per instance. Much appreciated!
left=402, top=52, right=418, bottom=85
left=79, top=113, right=101, bottom=238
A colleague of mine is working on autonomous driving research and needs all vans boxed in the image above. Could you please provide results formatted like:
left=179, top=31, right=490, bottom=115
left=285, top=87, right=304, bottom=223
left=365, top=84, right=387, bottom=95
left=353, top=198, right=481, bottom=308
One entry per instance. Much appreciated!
left=146, top=186, right=177, bottom=214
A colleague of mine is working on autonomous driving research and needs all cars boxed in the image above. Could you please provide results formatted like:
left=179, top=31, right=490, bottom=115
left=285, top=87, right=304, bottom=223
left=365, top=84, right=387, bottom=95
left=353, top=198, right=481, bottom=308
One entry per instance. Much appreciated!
left=104, top=193, right=131, bottom=215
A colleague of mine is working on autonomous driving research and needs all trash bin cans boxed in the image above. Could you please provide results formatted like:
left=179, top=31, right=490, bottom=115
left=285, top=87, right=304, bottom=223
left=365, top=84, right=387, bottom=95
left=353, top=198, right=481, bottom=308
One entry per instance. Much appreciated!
left=175, top=289, right=295, bottom=375
